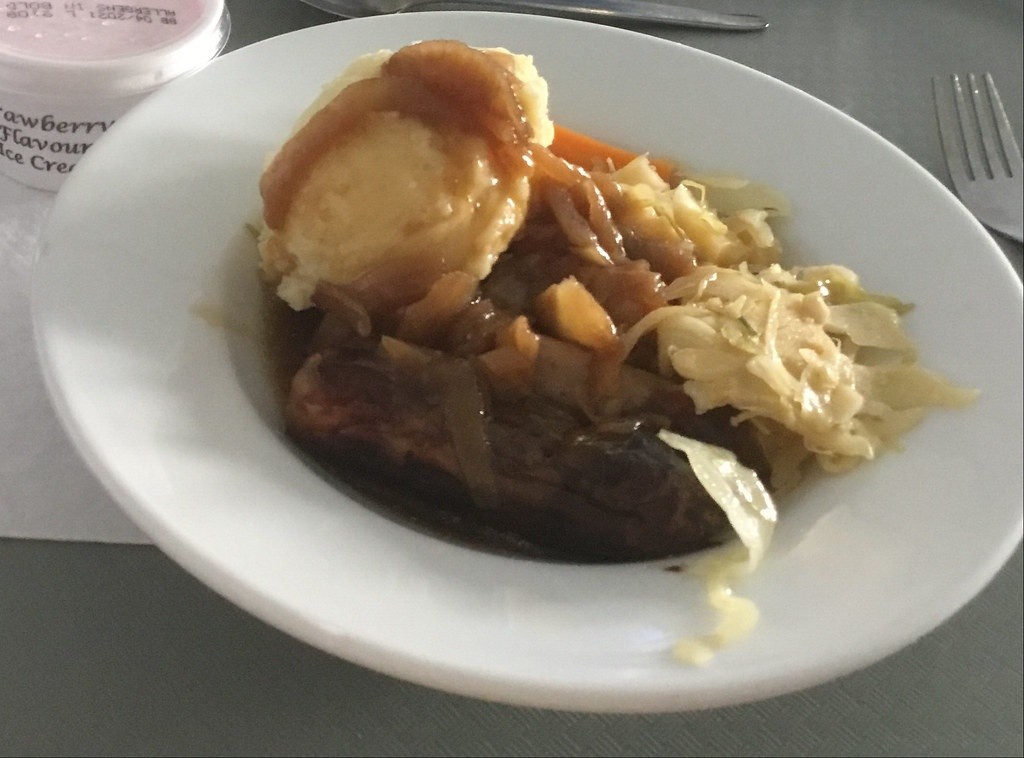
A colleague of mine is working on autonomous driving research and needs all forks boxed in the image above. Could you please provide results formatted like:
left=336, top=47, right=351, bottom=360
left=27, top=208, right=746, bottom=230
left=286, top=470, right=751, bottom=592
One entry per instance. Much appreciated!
left=927, top=66, right=1023, bottom=248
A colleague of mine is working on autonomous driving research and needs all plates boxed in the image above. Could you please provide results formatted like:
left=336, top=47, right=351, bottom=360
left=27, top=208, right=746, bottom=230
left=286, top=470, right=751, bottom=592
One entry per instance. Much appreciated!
left=27, top=10, right=1024, bottom=714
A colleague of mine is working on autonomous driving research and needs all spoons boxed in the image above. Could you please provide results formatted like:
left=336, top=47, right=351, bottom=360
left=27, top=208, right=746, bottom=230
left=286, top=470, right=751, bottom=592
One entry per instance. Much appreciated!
left=302, top=1, right=772, bottom=33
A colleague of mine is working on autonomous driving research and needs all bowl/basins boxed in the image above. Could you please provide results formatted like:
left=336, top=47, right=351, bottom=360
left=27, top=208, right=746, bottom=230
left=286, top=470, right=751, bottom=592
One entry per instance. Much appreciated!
left=1, top=1, right=234, bottom=193
